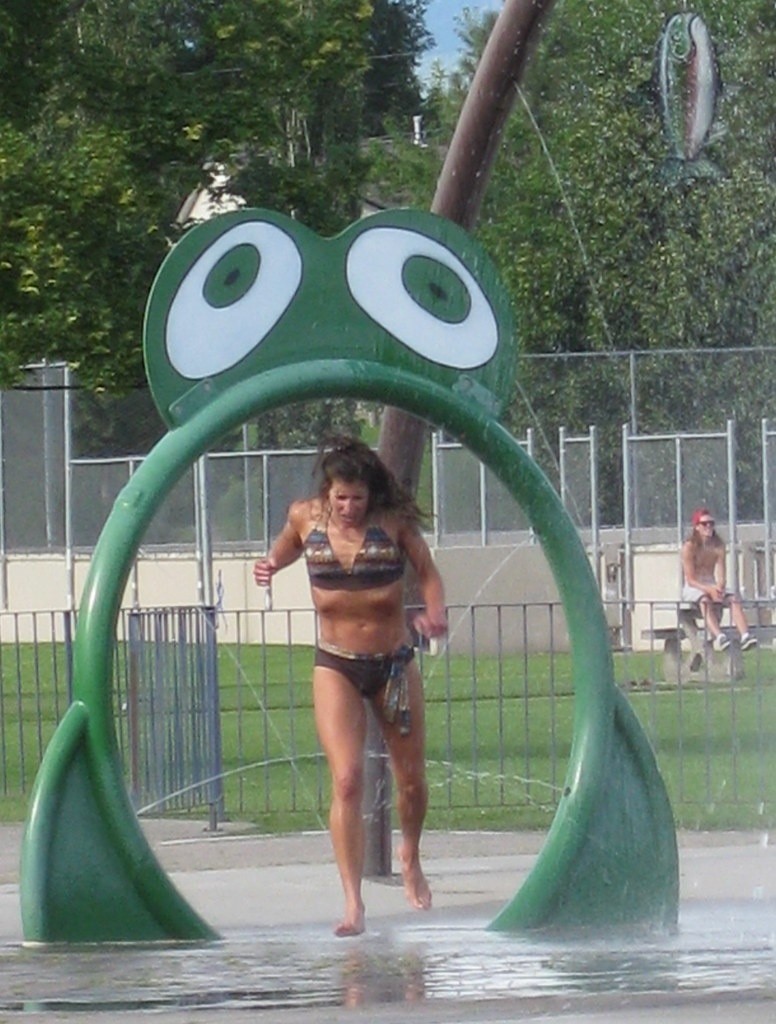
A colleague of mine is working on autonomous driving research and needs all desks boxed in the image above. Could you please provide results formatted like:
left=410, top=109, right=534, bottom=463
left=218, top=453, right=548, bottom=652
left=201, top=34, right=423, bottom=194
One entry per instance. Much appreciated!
left=651, top=598, right=776, bottom=684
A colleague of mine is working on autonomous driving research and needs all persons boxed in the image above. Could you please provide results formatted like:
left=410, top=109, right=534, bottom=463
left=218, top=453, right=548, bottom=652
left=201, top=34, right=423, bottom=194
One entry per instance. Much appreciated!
left=683, top=508, right=758, bottom=650
left=253, top=437, right=446, bottom=937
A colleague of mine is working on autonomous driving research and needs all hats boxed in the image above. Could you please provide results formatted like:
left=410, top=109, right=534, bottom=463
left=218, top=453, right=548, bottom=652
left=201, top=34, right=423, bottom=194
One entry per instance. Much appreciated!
left=692, top=509, right=714, bottom=524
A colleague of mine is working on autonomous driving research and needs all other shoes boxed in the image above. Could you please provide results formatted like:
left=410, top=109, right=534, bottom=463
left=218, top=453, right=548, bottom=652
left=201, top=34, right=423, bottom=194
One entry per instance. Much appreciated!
left=740, top=632, right=758, bottom=651
left=713, top=633, right=732, bottom=652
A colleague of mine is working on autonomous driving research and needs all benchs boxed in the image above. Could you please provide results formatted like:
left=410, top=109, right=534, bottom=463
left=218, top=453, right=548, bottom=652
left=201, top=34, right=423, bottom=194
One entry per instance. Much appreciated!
left=641, top=623, right=776, bottom=683
